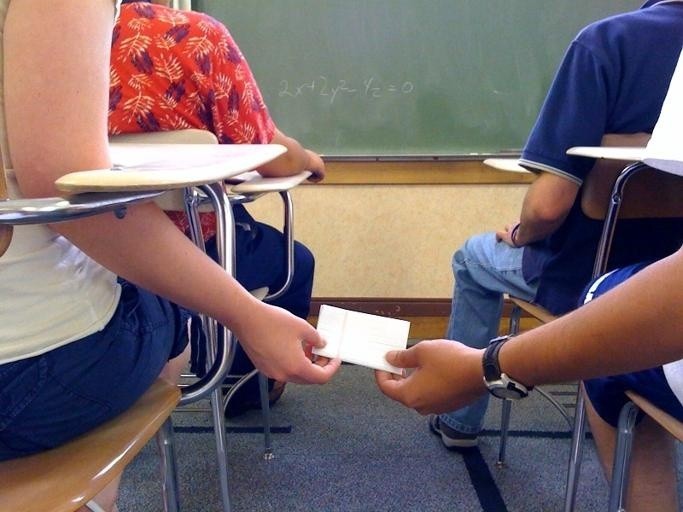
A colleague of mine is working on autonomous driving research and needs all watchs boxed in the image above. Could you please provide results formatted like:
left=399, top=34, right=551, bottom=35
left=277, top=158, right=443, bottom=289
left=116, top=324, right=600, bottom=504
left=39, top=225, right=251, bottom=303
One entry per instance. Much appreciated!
left=482, top=331, right=533, bottom=405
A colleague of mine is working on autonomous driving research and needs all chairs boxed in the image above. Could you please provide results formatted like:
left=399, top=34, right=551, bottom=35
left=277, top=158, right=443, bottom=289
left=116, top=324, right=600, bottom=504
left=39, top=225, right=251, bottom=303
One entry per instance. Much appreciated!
left=0, top=146, right=182, bottom=511
left=610, top=390, right=682, bottom=510
left=107, top=128, right=275, bottom=462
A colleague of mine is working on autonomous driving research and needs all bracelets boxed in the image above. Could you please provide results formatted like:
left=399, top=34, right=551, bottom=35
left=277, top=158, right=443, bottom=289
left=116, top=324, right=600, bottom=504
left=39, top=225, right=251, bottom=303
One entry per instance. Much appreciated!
left=511, top=223, right=521, bottom=247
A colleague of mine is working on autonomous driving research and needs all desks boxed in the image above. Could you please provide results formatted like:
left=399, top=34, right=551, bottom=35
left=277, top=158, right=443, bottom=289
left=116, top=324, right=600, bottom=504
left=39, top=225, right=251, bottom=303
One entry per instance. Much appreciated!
left=53, top=143, right=314, bottom=512
left=481, top=146, right=649, bottom=511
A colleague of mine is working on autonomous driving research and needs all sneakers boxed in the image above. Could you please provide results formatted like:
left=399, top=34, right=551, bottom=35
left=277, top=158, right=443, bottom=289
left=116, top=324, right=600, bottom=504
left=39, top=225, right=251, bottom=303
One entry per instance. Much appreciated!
left=430, top=416, right=478, bottom=449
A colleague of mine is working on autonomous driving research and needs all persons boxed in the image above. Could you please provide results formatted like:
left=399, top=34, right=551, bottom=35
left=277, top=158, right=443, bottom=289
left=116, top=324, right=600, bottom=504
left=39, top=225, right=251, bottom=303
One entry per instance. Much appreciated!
left=109, top=0, right=329, bottom=420
left=371, top=31, right=683, bottom=512
left=0, top=0, right=344, bottom=463
left=422, top=2, right=683, bottom=450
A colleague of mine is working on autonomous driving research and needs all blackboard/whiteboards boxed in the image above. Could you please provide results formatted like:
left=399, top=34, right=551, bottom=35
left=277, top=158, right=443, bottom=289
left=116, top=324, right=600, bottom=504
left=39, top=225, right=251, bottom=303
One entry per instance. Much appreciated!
left=170, top=2, right=646, bottom=161
